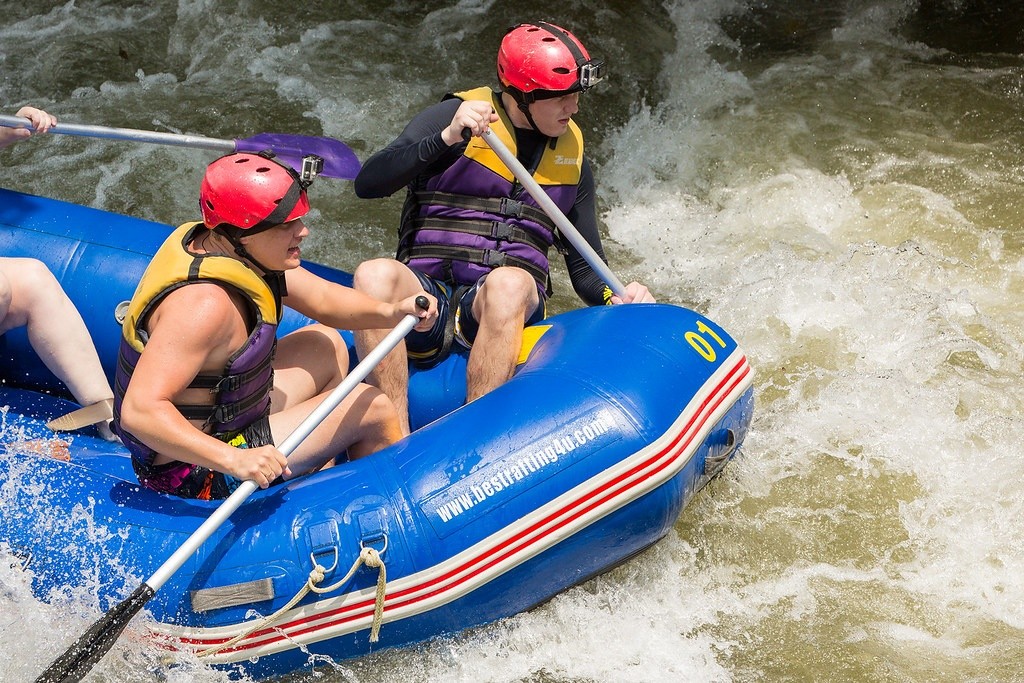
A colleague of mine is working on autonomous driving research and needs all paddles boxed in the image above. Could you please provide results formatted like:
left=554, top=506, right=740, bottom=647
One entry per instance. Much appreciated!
left=0, top=112, right=362, bottom=180
left=32, top=295, right=433, bottom=683
left=459, top=108, right=623, bottom=297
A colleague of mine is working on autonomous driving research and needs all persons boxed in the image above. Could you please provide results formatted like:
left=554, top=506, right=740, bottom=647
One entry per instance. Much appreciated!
left=0, top=105, right=119, bottom=443
left=116, top=154, right=441, bottom=498
left=354, top=25, right=657, bottom=437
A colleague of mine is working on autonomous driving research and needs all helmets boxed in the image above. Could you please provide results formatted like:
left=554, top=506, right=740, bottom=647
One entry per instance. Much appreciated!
left=497, top=21, right=592, bottom=93
left=202, top=151, right=309, bottom=230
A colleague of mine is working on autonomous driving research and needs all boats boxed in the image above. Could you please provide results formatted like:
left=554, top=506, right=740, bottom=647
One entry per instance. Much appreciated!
left=1, top=189, right=754, bottom=681
